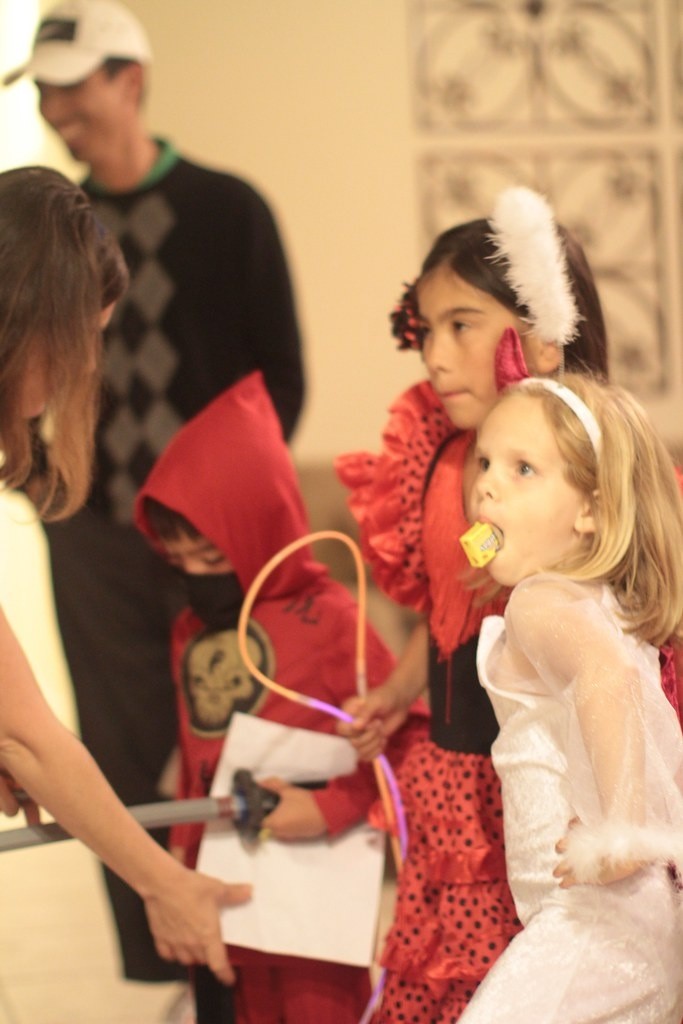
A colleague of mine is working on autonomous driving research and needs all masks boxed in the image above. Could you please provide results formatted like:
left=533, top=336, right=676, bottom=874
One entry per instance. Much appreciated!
left=167, top=570, right=244, bottom=631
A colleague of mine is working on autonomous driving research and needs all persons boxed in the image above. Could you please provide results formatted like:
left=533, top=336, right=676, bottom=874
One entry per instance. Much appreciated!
left=340, top=183, right=683, bottom=1023
left=1, top=0, right=311, bottom=1024
left=453, top=327, right=683, bottom=1023
left=132, top=368, right=420, bottom=1024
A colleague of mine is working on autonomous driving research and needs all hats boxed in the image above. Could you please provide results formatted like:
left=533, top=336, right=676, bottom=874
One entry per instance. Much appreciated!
left=3, top=0, right=147, bottom=84
